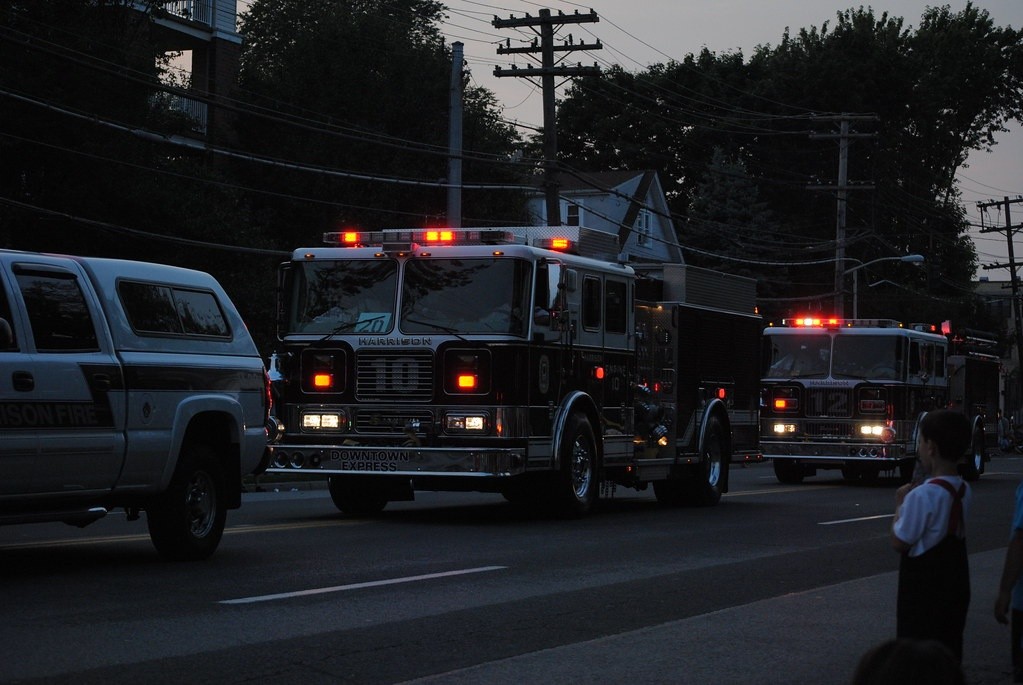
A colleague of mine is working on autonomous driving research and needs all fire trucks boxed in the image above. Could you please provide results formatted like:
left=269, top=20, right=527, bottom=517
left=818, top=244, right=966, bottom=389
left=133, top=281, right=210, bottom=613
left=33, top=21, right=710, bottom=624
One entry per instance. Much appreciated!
left=262, top=224, right=771, bottom=518
left=752, top=311, right=1004, bottom=486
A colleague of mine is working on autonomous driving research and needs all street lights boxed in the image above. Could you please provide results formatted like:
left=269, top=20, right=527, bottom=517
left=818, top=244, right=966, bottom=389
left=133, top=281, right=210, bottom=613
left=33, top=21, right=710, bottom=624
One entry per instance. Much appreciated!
left=831, top=253, right=925, bottom=327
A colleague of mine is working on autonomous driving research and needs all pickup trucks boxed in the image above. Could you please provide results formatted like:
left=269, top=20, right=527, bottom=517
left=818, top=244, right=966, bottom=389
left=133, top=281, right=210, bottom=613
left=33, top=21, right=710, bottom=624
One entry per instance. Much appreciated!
left=0, top=242, right=275, bottom=562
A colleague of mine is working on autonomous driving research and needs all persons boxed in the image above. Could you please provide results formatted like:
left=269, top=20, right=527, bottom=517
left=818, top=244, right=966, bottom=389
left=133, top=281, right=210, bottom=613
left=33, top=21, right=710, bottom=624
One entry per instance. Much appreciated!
left=991, top=477, right=1023, bottom=685
left=850, top=638, right=968, bottom=685
left=889, top=408, right=981, bottom=666
left=489, top=273, right=549, bottom=334
